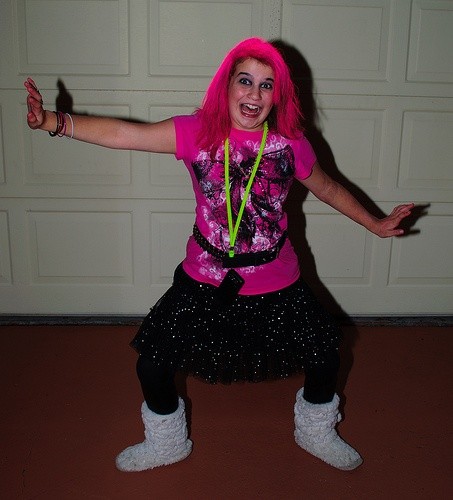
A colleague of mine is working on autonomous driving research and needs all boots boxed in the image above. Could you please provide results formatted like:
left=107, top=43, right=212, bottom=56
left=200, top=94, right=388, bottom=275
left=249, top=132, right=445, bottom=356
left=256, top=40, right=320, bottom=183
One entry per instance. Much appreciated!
left=115, top=396, right=193, bottom=472
left=293, top=387, right=364, bottom=470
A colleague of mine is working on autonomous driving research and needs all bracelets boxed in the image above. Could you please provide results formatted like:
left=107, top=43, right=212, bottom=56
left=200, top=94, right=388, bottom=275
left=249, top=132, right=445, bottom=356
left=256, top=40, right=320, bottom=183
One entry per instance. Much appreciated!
left=47, top=110, right=73, bottom=139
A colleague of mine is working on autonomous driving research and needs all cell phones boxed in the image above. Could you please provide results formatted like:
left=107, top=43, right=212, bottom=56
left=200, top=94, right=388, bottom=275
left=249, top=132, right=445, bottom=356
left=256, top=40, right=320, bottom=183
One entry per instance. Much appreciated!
left=215, top=269, right=243, bottom=307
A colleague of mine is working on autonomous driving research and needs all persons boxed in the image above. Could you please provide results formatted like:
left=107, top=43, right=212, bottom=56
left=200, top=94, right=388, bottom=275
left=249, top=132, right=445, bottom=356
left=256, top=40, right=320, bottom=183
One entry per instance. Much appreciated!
left=23, top=37, right=412, bottom=472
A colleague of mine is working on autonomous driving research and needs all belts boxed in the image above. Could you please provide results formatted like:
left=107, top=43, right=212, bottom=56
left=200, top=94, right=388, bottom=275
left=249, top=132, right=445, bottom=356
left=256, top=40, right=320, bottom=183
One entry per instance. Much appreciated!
left=192, top=223, right=288, bottom=268
